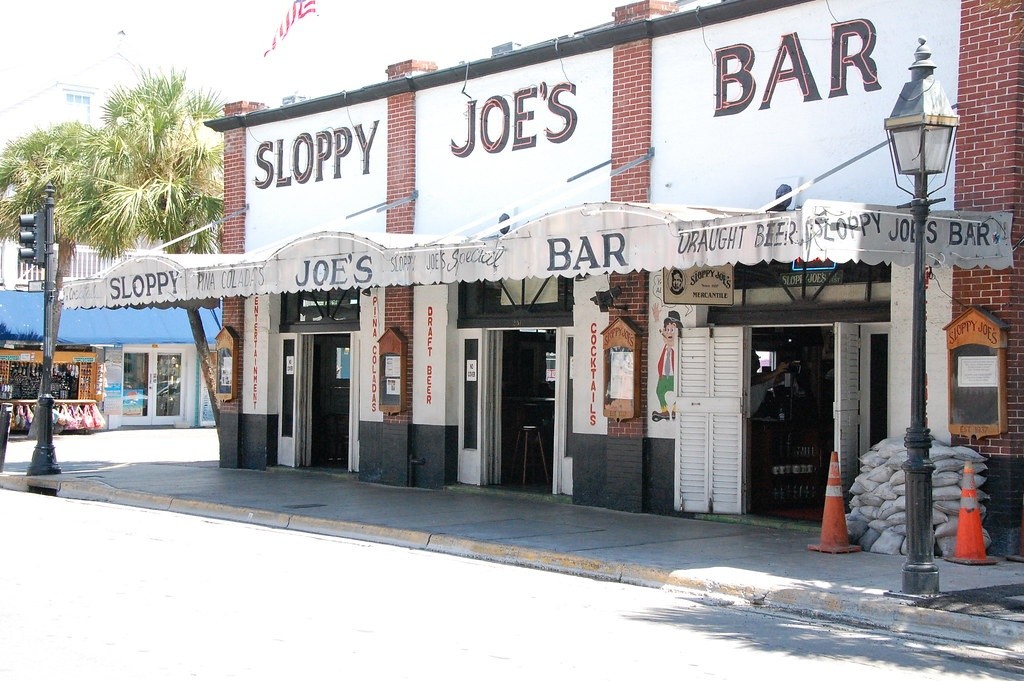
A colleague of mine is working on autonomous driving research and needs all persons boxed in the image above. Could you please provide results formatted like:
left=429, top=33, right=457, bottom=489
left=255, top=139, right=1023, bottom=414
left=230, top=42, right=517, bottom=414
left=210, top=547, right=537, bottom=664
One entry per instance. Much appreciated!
left=751, top=350, right=789, bottom=417
left=670, top=269, right=684, bottom=295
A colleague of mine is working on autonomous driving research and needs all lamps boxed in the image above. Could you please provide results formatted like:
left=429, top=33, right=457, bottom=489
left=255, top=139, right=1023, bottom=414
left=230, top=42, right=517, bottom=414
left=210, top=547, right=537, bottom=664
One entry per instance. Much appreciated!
left=589, top=285, right=629, bottom=313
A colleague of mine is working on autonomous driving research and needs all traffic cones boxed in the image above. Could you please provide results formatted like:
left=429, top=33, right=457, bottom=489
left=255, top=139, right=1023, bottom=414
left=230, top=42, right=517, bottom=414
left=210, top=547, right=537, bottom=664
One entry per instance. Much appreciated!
left=943, top=458, right=1002, bottom=568
left=806, top=450, right=863, bottom=552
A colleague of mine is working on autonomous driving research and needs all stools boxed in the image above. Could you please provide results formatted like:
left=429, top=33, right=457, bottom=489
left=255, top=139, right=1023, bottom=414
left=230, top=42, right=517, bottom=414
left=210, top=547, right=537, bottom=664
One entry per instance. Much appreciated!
left=509, top=425, right=549, bottom=486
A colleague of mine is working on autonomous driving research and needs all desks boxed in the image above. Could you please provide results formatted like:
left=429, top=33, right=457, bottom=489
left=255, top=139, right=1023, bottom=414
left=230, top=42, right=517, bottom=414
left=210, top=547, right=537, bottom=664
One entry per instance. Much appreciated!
left=752, top=418, right=823, bottom=512
left=0, top=399, right=97, bottom=434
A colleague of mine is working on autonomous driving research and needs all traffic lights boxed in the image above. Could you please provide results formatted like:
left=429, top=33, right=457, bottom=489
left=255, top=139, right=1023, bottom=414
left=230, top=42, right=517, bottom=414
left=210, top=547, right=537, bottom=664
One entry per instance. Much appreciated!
left=18, top=204, right=46, bottom=266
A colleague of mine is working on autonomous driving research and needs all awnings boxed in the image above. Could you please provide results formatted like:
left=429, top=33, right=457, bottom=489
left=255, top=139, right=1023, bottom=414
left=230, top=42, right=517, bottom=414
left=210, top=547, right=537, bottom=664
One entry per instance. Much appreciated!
left=0, top=290, right=223, bottom=345
left=58, top=104, right=1014, bottom=310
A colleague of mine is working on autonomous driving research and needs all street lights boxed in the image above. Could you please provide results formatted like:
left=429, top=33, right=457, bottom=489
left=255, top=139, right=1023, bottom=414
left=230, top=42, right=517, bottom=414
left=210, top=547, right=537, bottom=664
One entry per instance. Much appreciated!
left=882, top=37, right=961, bottom=608
